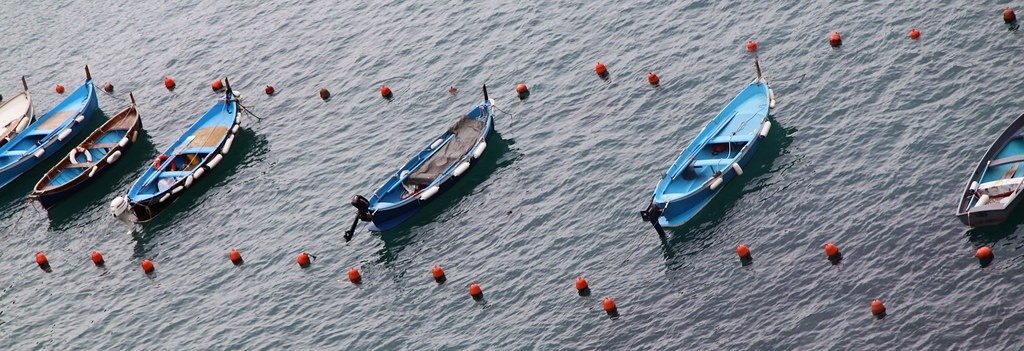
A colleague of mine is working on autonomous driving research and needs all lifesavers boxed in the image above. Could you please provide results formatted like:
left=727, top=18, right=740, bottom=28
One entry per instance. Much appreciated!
left=154, top=154, right=176, bottom=173
left=401, top=170, right=417, bottom=193
left=69, top=146, right=93, bottom=164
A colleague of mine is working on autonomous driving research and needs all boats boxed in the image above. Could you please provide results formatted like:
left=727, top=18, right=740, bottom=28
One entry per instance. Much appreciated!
left=342, top=85, right=496, bottom=243
left=640, top=59, right=776, bottom=242
left=0, top=67, right=98, bottom=198
left=0, top=75, right=34, bottom=149
left=109, top=78, right=240, bottom=233
left=955, top=109, right=1023, bottom=232
left=25, top=91, right=142, bottom=215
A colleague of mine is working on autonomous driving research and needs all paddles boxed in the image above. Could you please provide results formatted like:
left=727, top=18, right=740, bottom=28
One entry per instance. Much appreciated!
left=670, top=110, right=741, bottom=181
left=387, top=133, right=457, bottom=194
left=135, top=135, right=196, bottom=195
left=17, top=108, right=82, bottom=157
